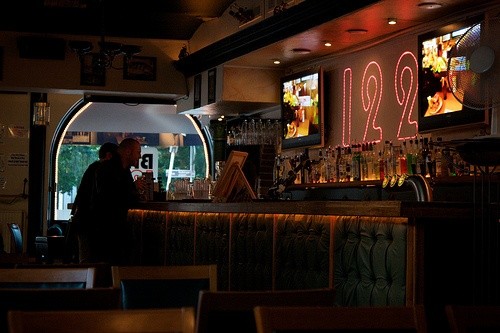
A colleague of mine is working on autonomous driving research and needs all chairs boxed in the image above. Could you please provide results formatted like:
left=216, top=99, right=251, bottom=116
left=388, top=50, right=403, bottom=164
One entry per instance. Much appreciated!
left=7, top=223, right=40, bottom=267
left=446, top=304, right=500, bottom=333
left=111, top=263, right=217, bottom=310
left=7, top=306, right=196, bottom=333
left=254, top=302, right=430, bottom=333
left=0, top=266, right=99, bottom=289
left=195, top=287, right=337, bottom=333
left=0, top=285, right=121, bottom=333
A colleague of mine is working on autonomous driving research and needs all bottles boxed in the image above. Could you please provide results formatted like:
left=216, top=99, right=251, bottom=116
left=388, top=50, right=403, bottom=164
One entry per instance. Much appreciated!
left=226, top=118, right=282, bottom=145
left=133, top=170, right=154, bottom=201
left=266, top=133, right=500, bottom=199
left=167, top=174, right=212, bottom=200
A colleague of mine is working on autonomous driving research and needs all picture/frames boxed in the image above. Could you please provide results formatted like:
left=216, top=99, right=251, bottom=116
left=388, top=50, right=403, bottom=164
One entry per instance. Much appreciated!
left=193, top=68, right=217, bottom=109
left=79, top=52, right=158, bottom=88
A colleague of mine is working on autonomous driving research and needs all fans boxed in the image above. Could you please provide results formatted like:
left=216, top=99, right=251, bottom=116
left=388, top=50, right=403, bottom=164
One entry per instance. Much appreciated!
left=446, top=15, right=500, bottom=143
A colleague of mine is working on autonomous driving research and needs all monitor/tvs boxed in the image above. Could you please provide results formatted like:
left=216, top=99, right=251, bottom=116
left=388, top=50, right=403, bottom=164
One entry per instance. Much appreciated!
left=417, top=13, right=491, bottom=134
left=279, top=65, right=329, bottom=153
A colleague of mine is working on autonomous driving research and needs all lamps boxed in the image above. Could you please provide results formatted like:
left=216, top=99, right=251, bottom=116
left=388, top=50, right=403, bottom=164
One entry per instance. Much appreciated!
left=31, top=95, right=51, bottom=129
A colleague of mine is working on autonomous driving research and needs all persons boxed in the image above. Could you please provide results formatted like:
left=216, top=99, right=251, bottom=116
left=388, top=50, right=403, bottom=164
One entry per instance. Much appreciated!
left=71, top=137, right=162, bottom=261
